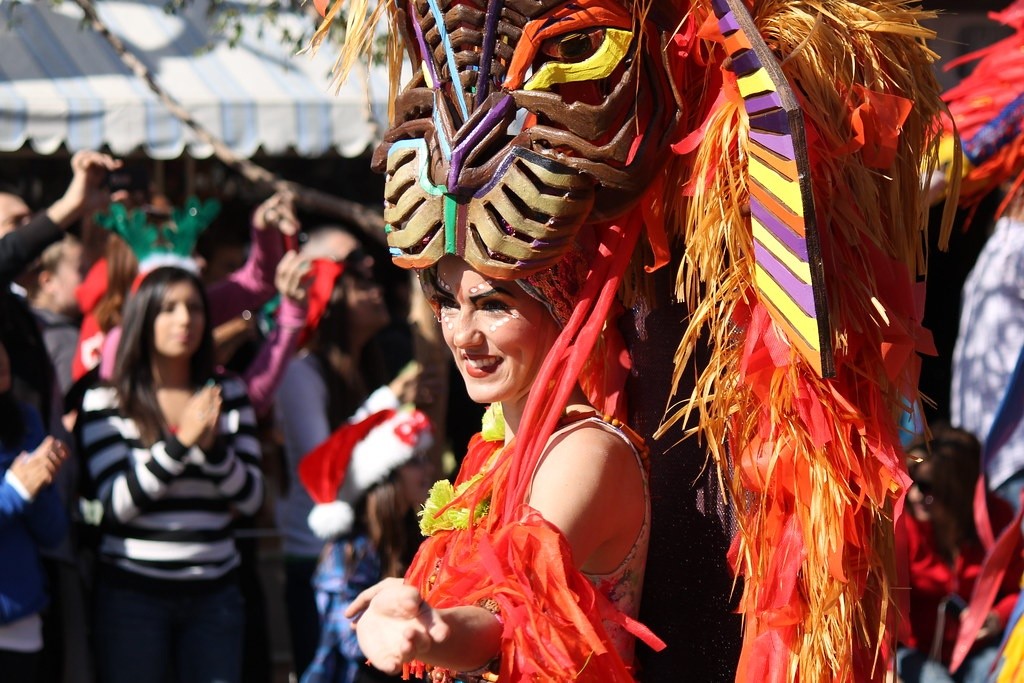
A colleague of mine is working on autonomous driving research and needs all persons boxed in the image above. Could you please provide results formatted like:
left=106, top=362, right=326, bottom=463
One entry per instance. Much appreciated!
left=347, top=251, right=651, bottom=683
left=890, top=427, right=1024, bottom=683
left=0, top=148, right=481, bottom=683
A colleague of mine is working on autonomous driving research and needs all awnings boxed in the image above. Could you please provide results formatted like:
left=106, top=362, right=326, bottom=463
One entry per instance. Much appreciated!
left=0, top=1, right=412, bottom=156
